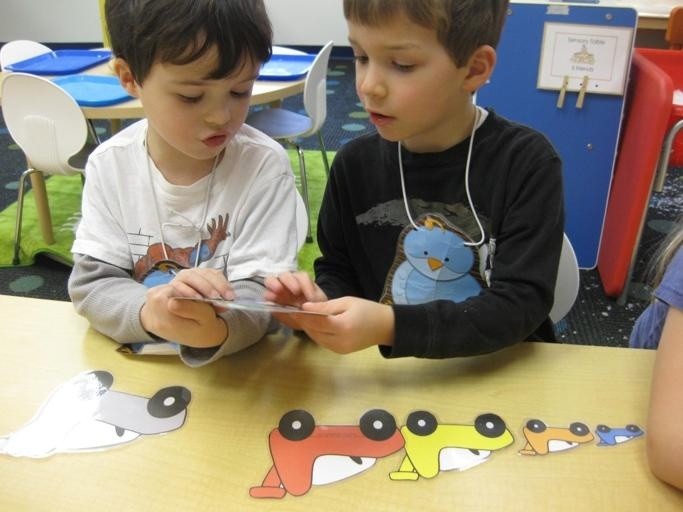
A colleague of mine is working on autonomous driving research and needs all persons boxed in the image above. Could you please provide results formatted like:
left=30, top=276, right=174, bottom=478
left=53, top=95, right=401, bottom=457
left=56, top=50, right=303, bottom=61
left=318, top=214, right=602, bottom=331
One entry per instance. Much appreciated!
left=628, top=224, right=683, bottom=490
left=65, top=1, right=309, bottom=372
left=264, top=1, right=564, bottom=359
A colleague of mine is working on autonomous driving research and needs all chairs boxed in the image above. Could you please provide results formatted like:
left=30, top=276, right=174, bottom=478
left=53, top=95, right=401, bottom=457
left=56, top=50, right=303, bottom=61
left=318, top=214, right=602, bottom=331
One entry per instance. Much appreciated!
left=1, top=71, right=99, bottom=265
left=246, top=39, right=334, bottom=244
left=1, top=39, right=53, bottom=72
left=549, top=232, right=582, bottom=325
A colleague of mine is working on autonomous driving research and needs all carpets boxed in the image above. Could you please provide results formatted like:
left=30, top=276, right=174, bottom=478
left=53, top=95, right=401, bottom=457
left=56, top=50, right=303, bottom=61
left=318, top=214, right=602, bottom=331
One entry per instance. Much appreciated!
left=0, top=151, right=337, bottom=280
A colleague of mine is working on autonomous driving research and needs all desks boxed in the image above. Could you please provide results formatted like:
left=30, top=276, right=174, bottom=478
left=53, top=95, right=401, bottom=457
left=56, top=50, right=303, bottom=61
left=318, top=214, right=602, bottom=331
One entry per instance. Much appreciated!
left=28, top=45, right=311, bottom=246
left=0, top=295, right=683, bottom=512
